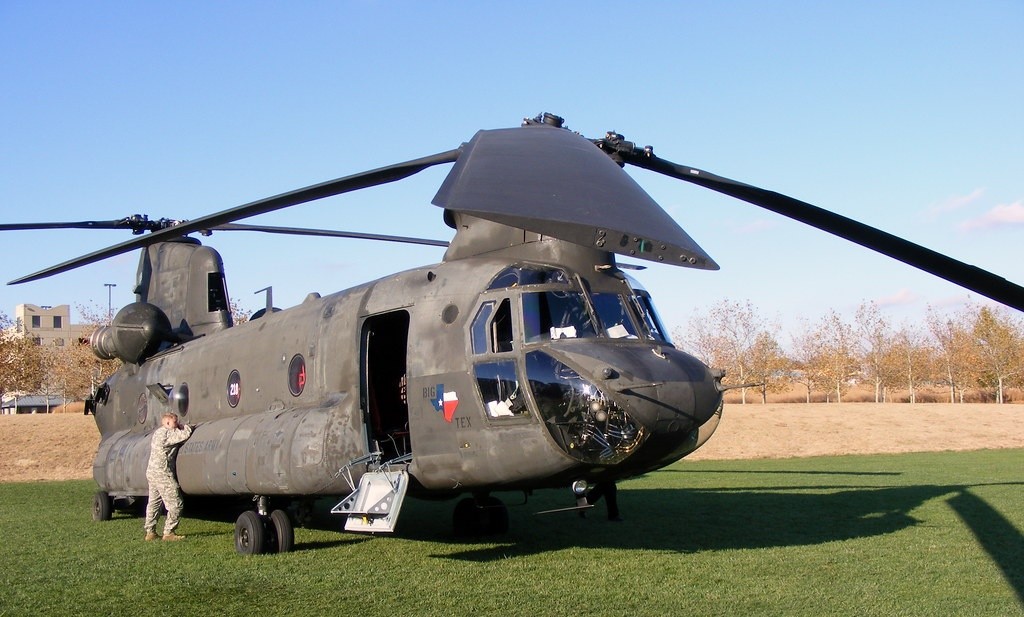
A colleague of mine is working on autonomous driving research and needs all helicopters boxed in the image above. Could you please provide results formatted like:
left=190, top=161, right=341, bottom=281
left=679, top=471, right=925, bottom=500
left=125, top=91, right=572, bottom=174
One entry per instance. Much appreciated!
left=2, top=110, right=1022, bottom=556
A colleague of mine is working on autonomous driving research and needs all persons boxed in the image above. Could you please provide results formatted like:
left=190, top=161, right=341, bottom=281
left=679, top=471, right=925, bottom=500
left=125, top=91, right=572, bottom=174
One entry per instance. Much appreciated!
left=144, top=413, right=196, bottom=541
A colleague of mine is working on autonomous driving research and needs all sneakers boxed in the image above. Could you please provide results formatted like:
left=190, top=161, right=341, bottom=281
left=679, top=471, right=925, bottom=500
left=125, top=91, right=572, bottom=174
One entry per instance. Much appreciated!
left=163, top=533, right=186, bottom=541
left=145, top=533, right=163, bottom=541
